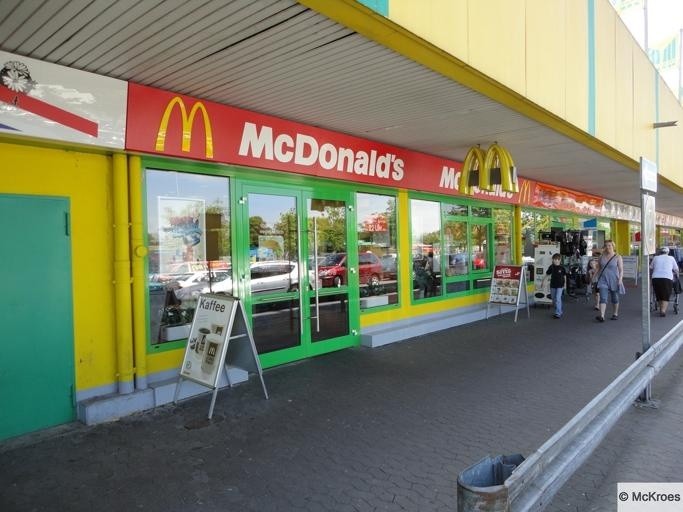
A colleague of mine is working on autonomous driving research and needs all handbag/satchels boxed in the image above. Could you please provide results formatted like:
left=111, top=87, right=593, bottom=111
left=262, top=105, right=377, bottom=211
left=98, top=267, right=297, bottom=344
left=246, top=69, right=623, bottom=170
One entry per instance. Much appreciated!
left=594, top=281, right=600, bottom=291
left=674, top=280, right=681, bottom=294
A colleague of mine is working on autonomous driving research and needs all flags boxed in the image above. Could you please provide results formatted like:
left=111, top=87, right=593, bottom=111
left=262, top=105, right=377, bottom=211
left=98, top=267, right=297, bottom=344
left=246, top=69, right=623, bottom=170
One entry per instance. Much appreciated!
left=635, top=232, right=640, bottom=241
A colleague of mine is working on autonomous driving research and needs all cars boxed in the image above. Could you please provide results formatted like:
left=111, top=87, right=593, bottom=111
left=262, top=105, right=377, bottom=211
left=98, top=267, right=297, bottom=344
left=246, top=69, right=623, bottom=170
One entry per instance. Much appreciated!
left=149, top=242, right=537, bottom=312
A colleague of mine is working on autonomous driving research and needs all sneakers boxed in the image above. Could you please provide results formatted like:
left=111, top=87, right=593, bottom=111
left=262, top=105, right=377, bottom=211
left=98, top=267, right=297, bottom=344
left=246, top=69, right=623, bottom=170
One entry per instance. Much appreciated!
left=596, top=315, right=604, bottom=321
left=660, top=311, right=665, bottom=317
left=610, top=315, right=618, bottom=320
left=594, top=305, right=599, bottom=311
left=552, top=314, right=562, bottom=318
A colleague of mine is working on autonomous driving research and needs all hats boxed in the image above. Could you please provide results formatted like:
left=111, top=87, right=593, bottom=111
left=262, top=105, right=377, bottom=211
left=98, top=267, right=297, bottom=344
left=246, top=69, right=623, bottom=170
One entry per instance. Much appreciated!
left=660, top=247, right=669, bottom=252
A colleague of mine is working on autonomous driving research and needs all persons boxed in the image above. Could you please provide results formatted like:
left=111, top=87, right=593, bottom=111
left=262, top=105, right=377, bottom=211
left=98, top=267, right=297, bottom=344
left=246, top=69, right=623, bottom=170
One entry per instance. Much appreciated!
left=588, top=260, right=600, bottom=311
left=541, top=253, right=567, bottom=319
left=591, top=239, right=623, bottom=321
left=413, top=244, right=484, bottom=299
left=650, top=246, right=680, bottom=317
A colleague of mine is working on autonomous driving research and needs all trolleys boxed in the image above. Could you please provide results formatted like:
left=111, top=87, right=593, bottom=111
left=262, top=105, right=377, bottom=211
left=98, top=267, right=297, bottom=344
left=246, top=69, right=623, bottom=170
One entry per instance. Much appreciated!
left=563, top=262, right=592, bottom=305
left=649, top=271, right=682, bottom=316
